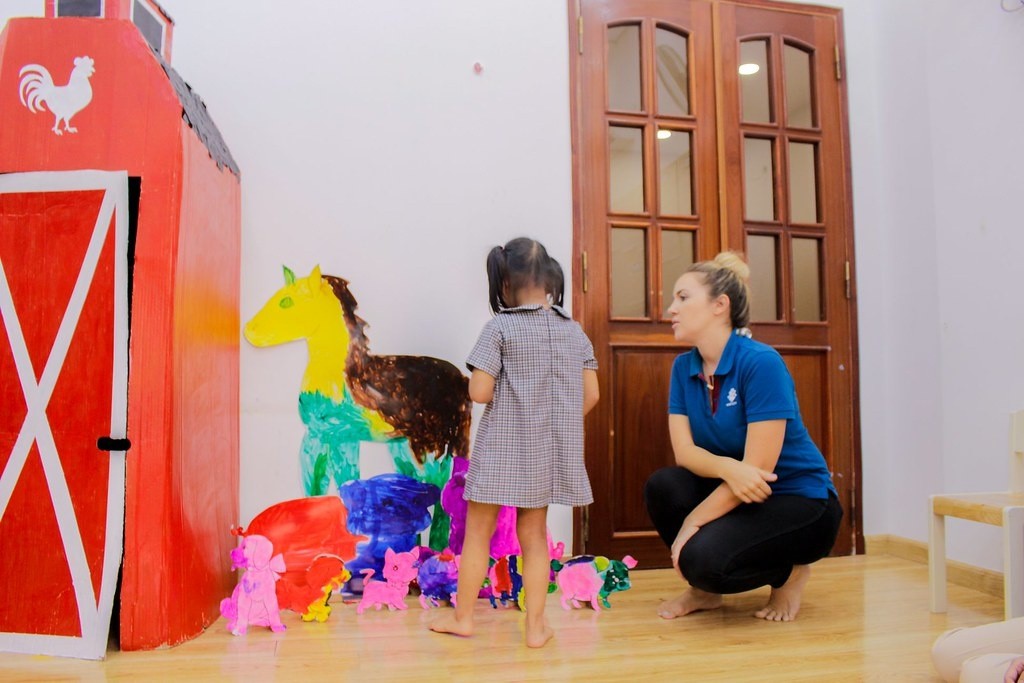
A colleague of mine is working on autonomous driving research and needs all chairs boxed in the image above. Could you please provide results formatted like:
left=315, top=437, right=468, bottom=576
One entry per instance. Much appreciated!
left=926, top=410, right=1024, bottom=620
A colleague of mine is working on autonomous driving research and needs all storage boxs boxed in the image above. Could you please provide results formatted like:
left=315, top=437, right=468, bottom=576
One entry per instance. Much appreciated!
left=44, top=0, right=177, bottom=69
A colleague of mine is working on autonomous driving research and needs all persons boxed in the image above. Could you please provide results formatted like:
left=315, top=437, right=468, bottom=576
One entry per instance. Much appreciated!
left=429, top=238, right=600, bottom=648
left=644, top=252, right=844, bottom=621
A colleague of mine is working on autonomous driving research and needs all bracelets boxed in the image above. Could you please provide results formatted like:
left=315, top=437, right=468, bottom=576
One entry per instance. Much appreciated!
left=671, top=556, right=673, bottom=558
left=755, top=489, right=760, bottom=494
